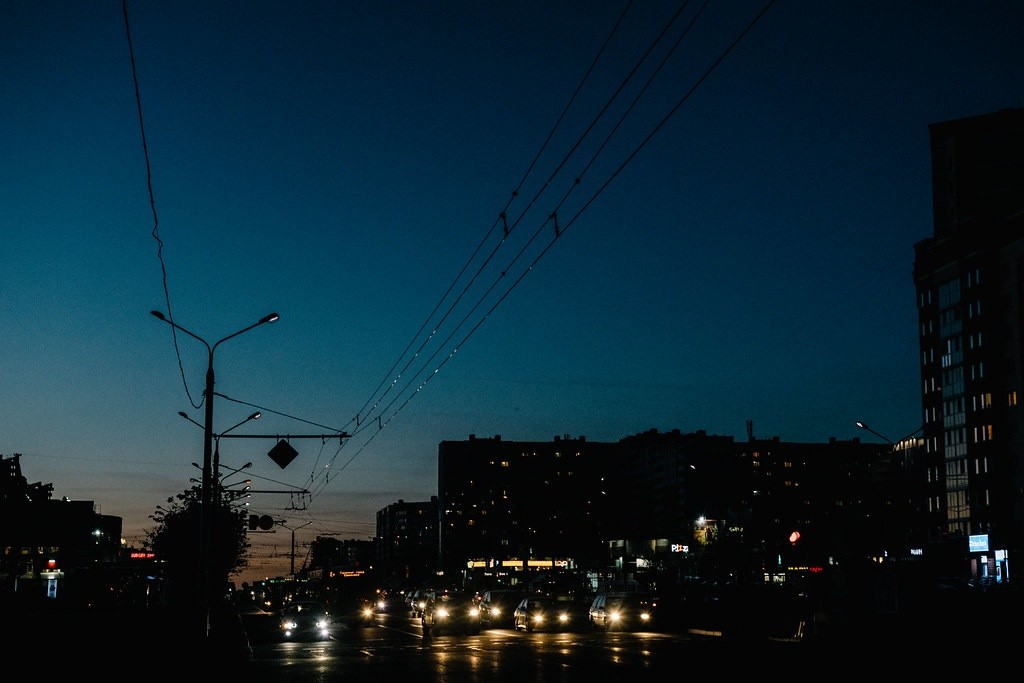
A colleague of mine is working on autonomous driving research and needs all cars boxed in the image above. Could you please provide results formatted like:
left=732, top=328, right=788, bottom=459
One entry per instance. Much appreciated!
left=238, top=546, right=1024, bottom=646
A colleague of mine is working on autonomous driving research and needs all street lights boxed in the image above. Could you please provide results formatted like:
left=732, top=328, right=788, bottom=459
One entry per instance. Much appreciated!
left=857, top=420, right=924, bottom=491
left=150, top=309, right=279, bottom=512
